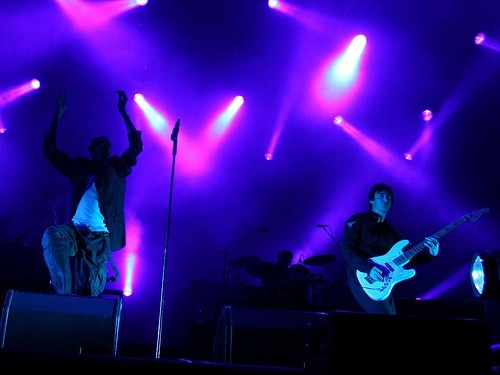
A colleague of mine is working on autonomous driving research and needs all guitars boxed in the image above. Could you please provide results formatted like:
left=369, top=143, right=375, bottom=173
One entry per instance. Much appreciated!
left=355, top=206, right=493, bottom=303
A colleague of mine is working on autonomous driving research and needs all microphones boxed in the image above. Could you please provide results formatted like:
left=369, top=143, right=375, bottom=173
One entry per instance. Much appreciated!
left=299, top=254, right=303, bottom=263
left=317, top=225, right=329, bottom=227
left=171, top=119, right=181, bottom=141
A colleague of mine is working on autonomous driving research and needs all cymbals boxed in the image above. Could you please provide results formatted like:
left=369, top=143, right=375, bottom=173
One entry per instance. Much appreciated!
left=302, top=253, right=337, bottom=266
left=232, top=256, right=262, bottom=268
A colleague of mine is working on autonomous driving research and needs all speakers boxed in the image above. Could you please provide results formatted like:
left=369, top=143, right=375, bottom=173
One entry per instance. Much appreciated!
left=0, top=289, right=123, bottom=358
left=212, top=307, right=492, bottom=375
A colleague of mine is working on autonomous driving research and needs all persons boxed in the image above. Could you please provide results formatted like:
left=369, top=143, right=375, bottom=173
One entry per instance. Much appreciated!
left=41, top=89, right=142, bottom=297
left=339, top=183, right=439, bottom=316
left=262, top=250, right=293, bottom=287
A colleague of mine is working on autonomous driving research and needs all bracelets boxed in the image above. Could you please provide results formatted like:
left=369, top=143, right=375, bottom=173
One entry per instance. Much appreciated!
left=121, top=115, right=129, bottom=120
